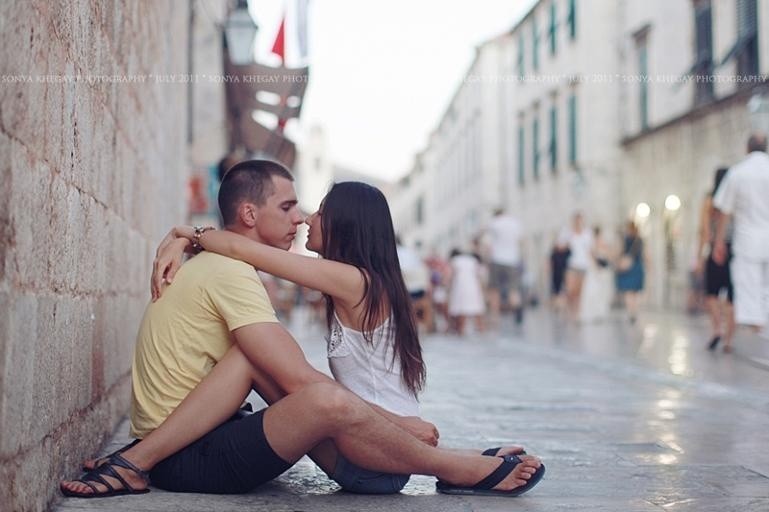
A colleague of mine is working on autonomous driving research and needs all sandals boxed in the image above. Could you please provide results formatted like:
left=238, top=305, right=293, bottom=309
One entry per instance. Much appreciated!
left=59, top=435, right=155, bottom=498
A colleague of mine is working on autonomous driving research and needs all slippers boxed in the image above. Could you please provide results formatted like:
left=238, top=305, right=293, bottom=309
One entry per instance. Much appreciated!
left=475, top=444, right=529, bottom=460
left=434, top=454, right=547, bottom=498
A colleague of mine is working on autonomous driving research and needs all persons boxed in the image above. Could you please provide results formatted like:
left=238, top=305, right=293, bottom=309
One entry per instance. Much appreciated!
left=393, top=204, right=650, bottom=333
left=60, top=183, right=422, bottom=498
left=698, top=168, right=736, bottom=353
left=256, top=267, right=325, bottom=320
left=715, top=135, right=769, bottom=336
left=128, top=161, right=546, bottom=494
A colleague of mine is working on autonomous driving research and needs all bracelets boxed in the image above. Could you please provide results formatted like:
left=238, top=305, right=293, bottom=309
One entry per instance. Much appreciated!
left=193, top=223, right=215, bottom=249
